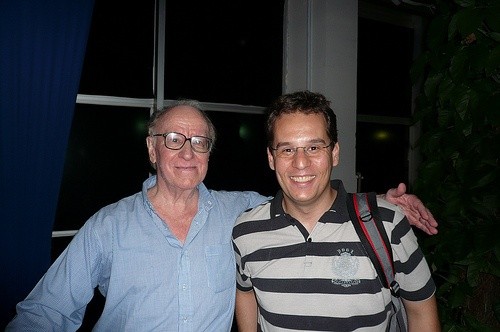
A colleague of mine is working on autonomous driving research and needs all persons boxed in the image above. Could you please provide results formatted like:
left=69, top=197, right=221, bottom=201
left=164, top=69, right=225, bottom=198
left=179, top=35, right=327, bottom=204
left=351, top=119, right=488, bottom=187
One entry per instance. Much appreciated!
left=4, top=101, right=439, bottom=331
left=232, top=90, right=440, bottom=332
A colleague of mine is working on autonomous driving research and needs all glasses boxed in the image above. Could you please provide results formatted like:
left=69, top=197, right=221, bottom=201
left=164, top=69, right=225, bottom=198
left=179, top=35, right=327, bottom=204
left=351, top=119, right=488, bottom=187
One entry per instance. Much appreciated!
left=271, top=142, right=332, bottom=156
left=153, top=132, right=213, bottom=153
